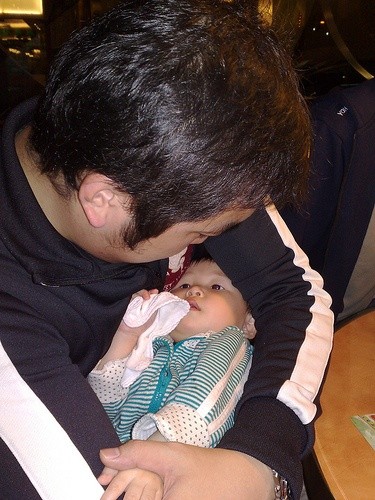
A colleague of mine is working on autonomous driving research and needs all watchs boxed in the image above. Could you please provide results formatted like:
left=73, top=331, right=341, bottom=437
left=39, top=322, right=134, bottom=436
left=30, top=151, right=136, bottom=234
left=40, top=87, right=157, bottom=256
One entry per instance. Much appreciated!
left=273, top=469, right=291, bottom=500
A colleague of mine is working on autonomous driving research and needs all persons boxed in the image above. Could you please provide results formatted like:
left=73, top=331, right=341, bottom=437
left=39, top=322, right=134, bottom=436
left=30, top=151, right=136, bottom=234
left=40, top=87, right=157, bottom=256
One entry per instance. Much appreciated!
left=0, top=0, right=335, bottom=500
left=85, top=242, right=256, bottom=500
left=286, top=76, right=375, bottom=326
